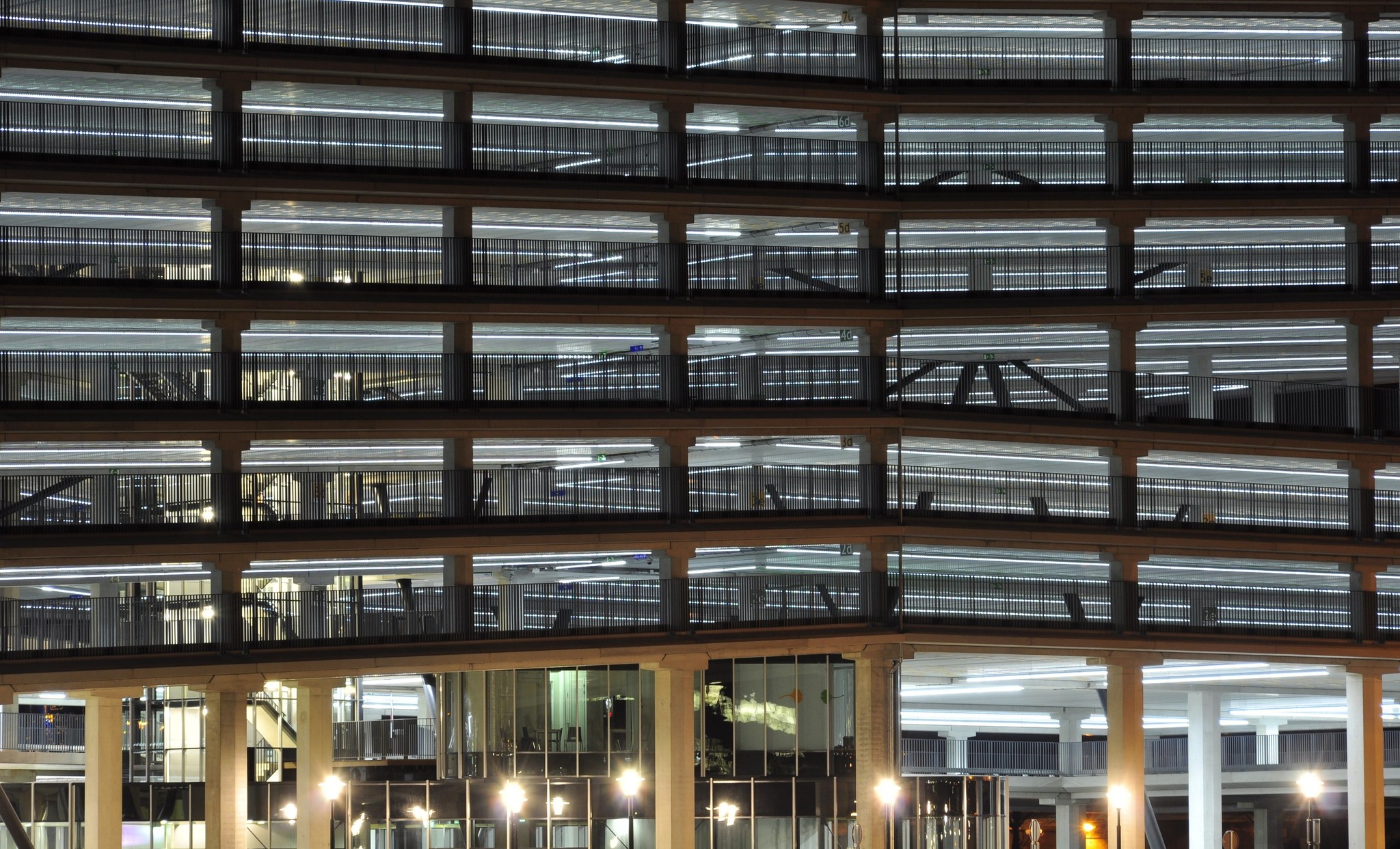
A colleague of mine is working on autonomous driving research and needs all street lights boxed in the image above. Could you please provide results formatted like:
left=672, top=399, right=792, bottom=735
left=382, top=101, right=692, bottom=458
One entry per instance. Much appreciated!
left=1298, top=771, right=1320, bottom=849
left=317, top=775, right=346, bottom=849
left=877, top=779, right=899, bottom=849
left=502, top=783, right=523, bottom=849
left=619, top=768, right=640, bottom=849
left=1107, top=784, right=1129, bottom=849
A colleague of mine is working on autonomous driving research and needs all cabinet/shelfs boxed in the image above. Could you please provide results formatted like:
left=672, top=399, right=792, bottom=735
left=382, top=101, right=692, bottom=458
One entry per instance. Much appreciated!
left=603, top=695, right=626, bottom=750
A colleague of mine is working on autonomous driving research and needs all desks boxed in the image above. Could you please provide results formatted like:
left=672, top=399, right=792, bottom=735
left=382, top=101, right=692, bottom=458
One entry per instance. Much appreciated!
left=534, top=729, right=562, bottom=752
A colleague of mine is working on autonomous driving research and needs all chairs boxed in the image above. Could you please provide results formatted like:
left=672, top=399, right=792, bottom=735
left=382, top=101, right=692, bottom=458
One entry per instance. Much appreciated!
left=637, top=726, right=653, bottom=751
left=499, top=726, right=584, bottom=752
